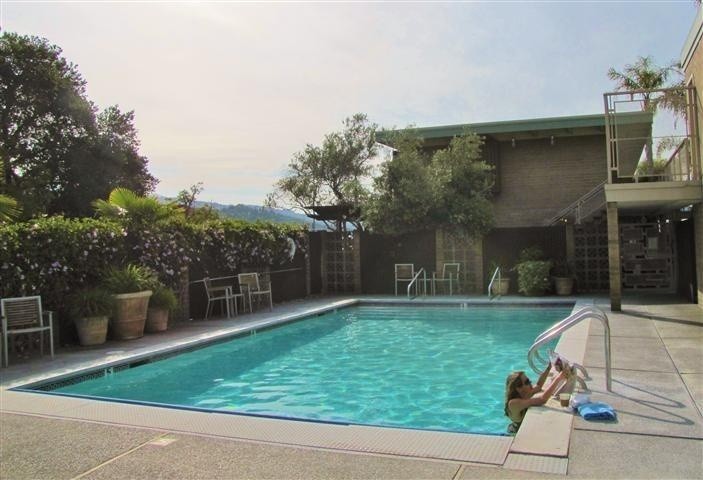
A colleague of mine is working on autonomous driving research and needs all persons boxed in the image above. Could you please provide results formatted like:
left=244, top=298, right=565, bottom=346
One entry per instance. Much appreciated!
left=503, top=360, right=571, bottom=433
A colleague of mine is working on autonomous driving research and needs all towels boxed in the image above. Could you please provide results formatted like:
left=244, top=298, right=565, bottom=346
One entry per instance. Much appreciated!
left=577, top=401, right=615, bottom=420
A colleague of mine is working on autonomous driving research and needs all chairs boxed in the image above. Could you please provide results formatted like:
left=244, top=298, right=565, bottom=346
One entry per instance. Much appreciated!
left=0, top=295, right=54, bottom=368
left=393, top=262, right=460, bottom=297
left=201, top=272, right=273, bottom=319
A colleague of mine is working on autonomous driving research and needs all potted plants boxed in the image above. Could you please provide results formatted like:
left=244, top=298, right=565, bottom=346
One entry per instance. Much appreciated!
left=71, top=265, right=175, bottom=347
left=488, top=245, right=574, bottom=296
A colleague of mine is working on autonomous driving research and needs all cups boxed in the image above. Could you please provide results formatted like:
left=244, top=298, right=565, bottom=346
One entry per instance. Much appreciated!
left=559, top=393, right=570, bottom=408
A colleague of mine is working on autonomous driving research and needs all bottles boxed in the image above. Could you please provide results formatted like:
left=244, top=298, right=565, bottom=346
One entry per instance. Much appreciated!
left=545, top=348, right=577, bottom=379
